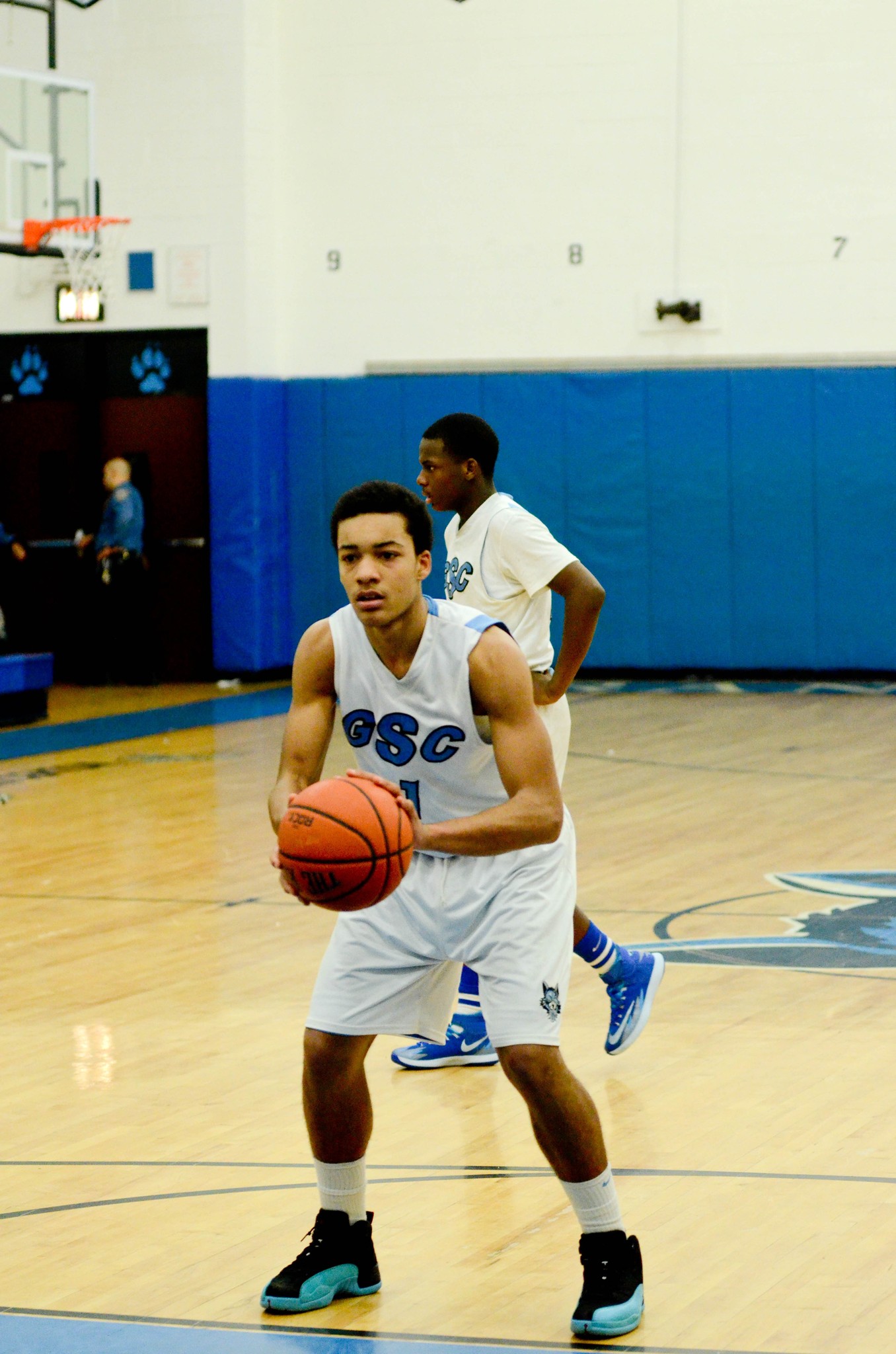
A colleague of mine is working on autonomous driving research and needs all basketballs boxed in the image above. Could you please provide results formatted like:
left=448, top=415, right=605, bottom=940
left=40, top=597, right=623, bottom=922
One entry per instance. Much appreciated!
left=277, top=772, right=415, bottom=908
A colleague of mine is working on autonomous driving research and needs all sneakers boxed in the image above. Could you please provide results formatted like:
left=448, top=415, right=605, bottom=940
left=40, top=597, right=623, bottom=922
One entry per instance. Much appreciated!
left=391, top=1025, right=498, bottom=1069
left=571, top=1231, right=644, bottom=1337
left=261, top=1207, right=381, bottom=1312
left=599, top=945, right=664, bottom=1055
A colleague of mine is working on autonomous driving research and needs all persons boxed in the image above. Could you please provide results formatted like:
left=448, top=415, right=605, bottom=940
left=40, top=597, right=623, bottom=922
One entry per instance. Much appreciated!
left=0, top=520, right=27, bottom=639
left=262, top=483, right=649, bottom=1343
left=393, top=412, right=666, bottom=1070
left=80, top=456, right=150, bottom=687
